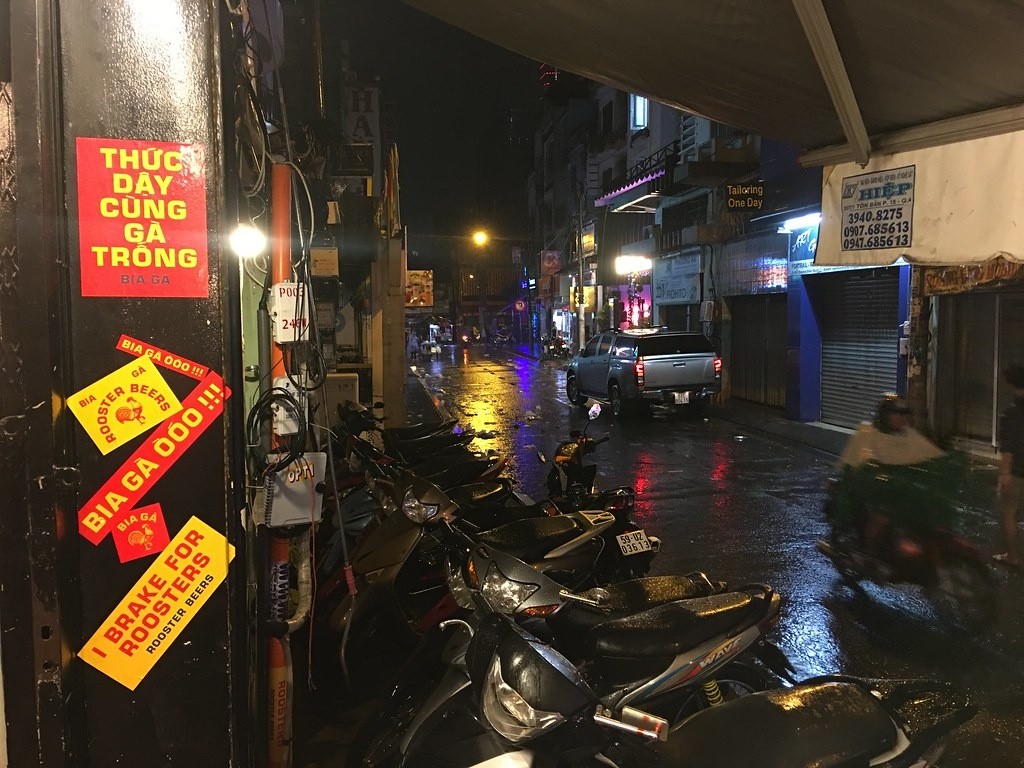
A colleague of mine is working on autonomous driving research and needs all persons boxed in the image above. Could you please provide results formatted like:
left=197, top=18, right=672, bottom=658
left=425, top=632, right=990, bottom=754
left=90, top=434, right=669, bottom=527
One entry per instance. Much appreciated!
left=992, top=365, right=1024, bottom=565
left=408, top=330, right=419, bottom=359
left=830, top=391, right=946, bottom=558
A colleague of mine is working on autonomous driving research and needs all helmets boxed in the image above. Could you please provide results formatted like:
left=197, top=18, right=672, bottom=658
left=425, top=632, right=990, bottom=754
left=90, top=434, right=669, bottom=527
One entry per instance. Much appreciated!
left=877, top=393, right=911, bottom=415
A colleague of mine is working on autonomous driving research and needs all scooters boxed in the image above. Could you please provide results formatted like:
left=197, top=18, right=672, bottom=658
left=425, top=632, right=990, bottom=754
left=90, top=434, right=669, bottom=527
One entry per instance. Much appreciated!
left=819, top=456, right=996, bottom=640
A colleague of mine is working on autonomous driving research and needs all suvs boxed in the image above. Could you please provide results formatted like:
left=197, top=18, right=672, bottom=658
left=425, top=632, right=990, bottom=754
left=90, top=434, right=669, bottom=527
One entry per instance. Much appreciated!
left=567, top=325, right=723, bottom=424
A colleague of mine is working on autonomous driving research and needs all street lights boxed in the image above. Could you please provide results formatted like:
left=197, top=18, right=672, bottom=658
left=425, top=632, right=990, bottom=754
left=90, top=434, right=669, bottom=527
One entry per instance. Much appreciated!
left=469, top=230, right=533, bottom=348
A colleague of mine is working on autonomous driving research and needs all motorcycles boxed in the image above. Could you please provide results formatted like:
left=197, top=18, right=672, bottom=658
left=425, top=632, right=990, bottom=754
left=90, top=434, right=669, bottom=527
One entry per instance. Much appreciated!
left=541, top=333, right=574, bottom=362
left=292, top=398, right=981, bottom=768
